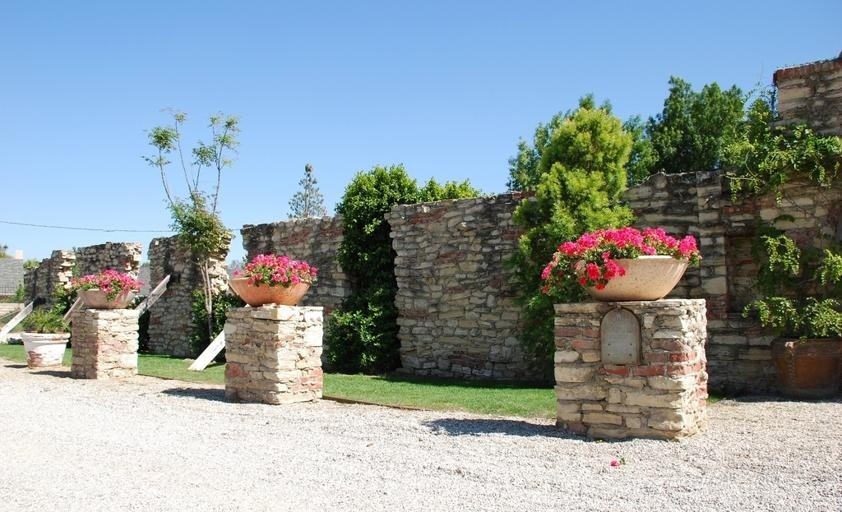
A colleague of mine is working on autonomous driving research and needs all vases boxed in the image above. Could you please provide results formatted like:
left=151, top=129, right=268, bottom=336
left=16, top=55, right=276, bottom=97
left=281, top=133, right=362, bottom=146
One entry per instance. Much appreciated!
left=227, top=273, right=312, bottom=307
left=579, top=252, right=692, bottom=302
left=76, top=286, right=140, bottom=308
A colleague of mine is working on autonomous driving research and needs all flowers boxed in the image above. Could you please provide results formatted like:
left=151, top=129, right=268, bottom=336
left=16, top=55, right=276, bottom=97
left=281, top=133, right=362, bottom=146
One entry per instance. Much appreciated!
left=78, top=268, right=147, bottom=302
left=228, top=251, right=321, bottom=289
left=538, top=225, right=705, bottom=303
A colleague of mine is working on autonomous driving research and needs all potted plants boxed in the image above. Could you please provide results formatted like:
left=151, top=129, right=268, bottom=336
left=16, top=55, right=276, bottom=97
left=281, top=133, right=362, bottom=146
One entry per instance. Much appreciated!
left=17, top=306, right=72, bottom=372
left=737, top=221, right=841, bottom=397
